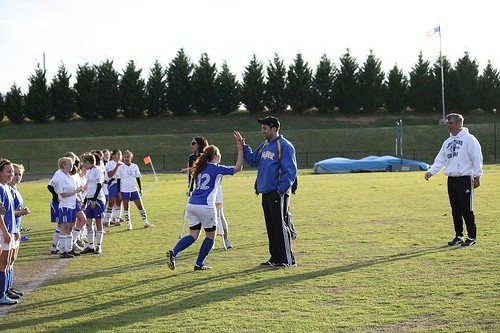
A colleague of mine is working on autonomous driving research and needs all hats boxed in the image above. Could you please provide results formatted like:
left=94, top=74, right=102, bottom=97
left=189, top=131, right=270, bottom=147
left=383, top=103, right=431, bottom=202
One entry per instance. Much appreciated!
left=257, top=116, right=281, bottom=126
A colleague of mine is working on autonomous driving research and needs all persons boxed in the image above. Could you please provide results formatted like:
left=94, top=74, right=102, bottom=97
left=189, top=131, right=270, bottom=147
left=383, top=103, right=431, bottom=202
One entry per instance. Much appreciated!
left=166, top=137, right=244, bottom=270
left=56, top=157, right=80, bottom=258
left=81, top=155, right=106, bottom=254
left=47, top=150, right=125, bottom=252
left=117, top=151, right=155, bottom=230
left=0, top=159, right=32, bottom=304
left=423, top=114, right=483, bottom=246
left=234, top=116, right=298, bottom=267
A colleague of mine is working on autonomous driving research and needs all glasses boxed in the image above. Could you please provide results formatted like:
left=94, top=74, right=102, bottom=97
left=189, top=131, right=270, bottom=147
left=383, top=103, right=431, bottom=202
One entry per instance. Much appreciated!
left=192, top=141, right=197, bottom=146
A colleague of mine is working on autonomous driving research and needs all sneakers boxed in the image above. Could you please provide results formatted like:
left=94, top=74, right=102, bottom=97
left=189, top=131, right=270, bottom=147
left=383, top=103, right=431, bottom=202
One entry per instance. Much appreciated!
left=461, top=239, right=477, bottom=248
left=447, top=237, right=465, bottom=245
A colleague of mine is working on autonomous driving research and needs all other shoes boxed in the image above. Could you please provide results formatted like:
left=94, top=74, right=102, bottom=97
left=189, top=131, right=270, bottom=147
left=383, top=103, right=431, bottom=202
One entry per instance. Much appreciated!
left=260, top=262, right=273, bottom=267
left=68, top=250, right=80, bottom=256
left=73, top=243, right=82, bottom=251
left=95, top=247, right=103, bottom=254
left=11, top=288, right=24, bottom=296
left=127, top=225, right=133, bottom=230
left=80, top=247, right=95, bottom=254
left=0, top=296, right=17, bottom=305
left=59, top=252, right=74, bottom=258
left=273, top=262, right=292, bottom=269
left=115, top=221, right=122, bottom=226
left=51, top=249, right=60, bottom=254
left=76, top=237, right=89, bottom=247
left=166, top=249, right=176, bottom=270
left=194, top=264, right=213, bottom=270
left=224, top=239, right=233, bottom=250
left=120, top=218, right=124, bottom=222
left=144, top=224, right=153, bottom=228
left=7, top=290, right=20, bottom=298
